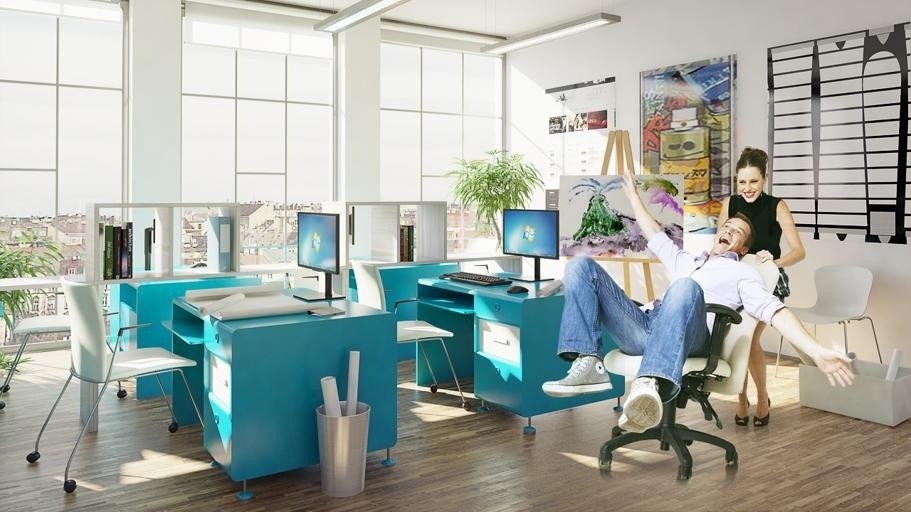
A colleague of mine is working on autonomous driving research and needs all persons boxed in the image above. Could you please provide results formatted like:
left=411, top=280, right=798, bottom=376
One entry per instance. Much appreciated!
left=707, top=146, right=807, bottom=427
left=541, top=166, right=858, bottom=433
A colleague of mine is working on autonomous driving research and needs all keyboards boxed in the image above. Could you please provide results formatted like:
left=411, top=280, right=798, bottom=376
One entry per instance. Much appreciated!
left=439, top=272, right=512, bottom=286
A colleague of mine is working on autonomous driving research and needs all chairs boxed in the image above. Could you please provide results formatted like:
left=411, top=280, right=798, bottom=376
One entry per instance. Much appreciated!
left=1, top=274, right=92, bottom=395
left=773, top=265, right=883, bottom=370
left=25, top=274, right=204, bottom=492
left=596, top=250, right=788, bottom=481
left=349, top=256, right=473, bottom=414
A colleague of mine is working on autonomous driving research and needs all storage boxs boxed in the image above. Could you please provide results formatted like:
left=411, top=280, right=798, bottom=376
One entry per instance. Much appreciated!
left=794, top=353, right=910, bottom=429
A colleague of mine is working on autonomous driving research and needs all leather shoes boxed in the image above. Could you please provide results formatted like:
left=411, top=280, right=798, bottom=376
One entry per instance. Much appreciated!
left=735, top=398, right=750, bottom=425
left=753, top=397, right=770, bottom=427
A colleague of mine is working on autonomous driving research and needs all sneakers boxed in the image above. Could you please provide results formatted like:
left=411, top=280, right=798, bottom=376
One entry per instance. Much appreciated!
left=541, top=355, right=613, bottom=398
left=618, top=375, right=663, bottom=433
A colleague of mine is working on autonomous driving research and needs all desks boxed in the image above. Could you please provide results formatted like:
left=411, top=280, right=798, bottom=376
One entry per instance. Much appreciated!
left=348, top=251, right=629, bottom=439
left=105, top=270, right=400, bottom=502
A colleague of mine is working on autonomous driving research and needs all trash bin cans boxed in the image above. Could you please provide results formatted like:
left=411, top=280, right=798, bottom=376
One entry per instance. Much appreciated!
left=316, top=400, right=370, bottom=496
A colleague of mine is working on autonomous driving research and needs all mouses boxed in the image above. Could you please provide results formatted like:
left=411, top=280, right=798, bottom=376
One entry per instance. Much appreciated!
left=507, top=286, right=529, bottom=293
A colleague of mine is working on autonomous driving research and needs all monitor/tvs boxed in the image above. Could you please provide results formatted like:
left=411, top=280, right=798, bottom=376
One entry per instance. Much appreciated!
left=293, top=212, right=346, bottom=303
left=503, top=208, right=560, bottom=282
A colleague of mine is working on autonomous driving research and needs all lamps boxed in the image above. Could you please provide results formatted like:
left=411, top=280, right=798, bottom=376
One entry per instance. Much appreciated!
left=480, top=10, right=623, bottom=62
left=311, top=0, right=413, bottom=36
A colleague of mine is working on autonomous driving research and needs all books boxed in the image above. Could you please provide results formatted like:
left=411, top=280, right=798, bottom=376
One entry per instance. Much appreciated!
left=120, top=228, right=128, bottom=278
left=104, top=224, right=114, bottom=280
left=99, top=217, right=106, bottom=282
left=113, top=226, right=123, bottom=279
left=400, top=224, right=415, bottom=262
left=127, top=221, right=132, bottom=279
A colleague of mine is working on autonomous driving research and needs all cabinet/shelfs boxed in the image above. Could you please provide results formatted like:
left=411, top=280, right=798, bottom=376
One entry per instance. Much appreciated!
left=85, top=201, right=241, bottom=282
left=314, top=194, right=448, bottom=266
